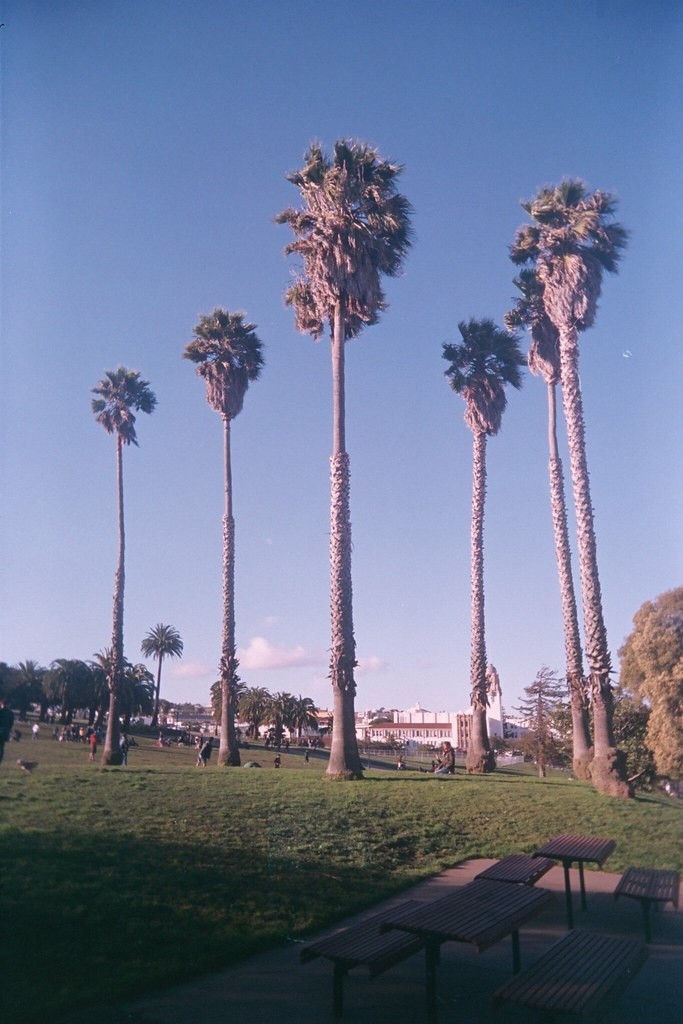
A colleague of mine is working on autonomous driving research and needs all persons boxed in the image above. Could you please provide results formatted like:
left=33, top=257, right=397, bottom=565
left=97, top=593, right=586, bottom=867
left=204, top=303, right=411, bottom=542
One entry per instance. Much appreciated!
left=434, top=741, right=455, bottom=775
left=0, top=699, right=314, bottom=768
left=419, top=750, right=445, bottom=772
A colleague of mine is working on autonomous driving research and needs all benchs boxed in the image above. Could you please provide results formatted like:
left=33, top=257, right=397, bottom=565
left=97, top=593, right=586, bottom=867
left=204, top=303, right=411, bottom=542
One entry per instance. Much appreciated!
left=492, top=929, right=650, bottom=1024
left=299, top=899, right=448, bottom=1024
left=614, top=866, right=680, bottom=944
left=474, top=854, right=554, bottom=887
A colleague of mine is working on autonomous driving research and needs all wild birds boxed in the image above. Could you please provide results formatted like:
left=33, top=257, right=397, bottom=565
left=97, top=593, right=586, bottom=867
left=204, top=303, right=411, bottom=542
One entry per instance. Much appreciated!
left=17, top=759, right=39, bottom=774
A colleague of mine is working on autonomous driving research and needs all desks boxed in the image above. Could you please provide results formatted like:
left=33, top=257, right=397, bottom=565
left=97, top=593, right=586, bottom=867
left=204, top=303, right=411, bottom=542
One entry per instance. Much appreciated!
left=532, top=835, right=617, bottom=930
left=379, top=878, right=554, bottom=1024
